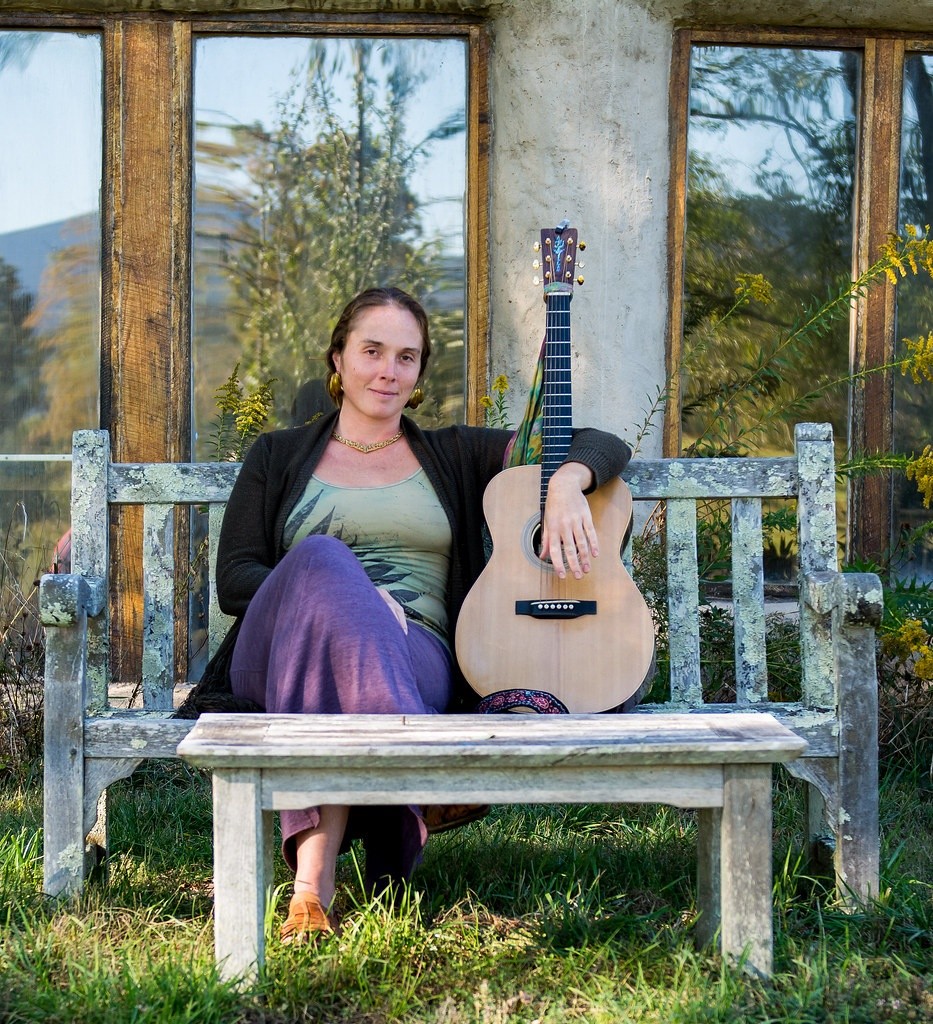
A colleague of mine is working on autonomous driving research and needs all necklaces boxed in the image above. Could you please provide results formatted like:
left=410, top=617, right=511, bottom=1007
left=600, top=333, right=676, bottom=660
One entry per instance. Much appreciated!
left=332, top=431, right=403, bottom=452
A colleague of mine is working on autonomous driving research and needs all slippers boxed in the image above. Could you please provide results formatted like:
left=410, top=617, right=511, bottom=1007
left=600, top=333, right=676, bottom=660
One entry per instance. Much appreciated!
left=281, top=890, right=342, bottom=950
left=421, top=804, right=492, bottom=835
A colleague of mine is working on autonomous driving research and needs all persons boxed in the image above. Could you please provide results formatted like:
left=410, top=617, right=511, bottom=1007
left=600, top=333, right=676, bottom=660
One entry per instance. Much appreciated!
left=216, top=284, right=632, bottom=945
left=291, top=378, right=335, bottom=427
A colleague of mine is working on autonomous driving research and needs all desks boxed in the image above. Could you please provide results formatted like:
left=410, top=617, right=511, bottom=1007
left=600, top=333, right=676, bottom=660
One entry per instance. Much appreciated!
left=174, top=704, right=813, bottom=994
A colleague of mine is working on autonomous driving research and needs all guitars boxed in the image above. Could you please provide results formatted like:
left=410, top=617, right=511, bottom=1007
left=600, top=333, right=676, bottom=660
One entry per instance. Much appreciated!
left=453, top=222, right=661, bottom=718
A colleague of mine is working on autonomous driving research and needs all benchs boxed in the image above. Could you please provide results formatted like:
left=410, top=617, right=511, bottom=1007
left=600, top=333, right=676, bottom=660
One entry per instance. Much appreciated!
left=32, top=419, right=892, bottom=911
